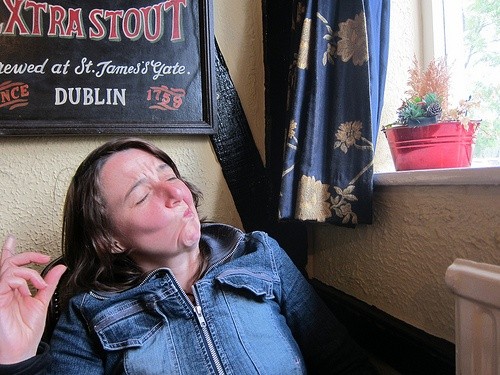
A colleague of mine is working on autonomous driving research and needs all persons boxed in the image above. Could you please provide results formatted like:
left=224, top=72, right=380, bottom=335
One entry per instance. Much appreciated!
left=0, top=138, right=353, bottom=375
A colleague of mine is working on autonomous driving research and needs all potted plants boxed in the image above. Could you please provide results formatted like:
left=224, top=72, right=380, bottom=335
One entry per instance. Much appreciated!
left=381, top=120, right=483, bottom=171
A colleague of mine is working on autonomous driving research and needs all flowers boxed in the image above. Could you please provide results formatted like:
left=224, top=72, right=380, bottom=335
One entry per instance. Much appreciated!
left=382, top=52, right=482, bottom=132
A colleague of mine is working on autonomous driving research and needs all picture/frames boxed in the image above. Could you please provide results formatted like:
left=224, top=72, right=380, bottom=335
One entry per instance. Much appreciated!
left=0, top=0, right=219, bottom=137
